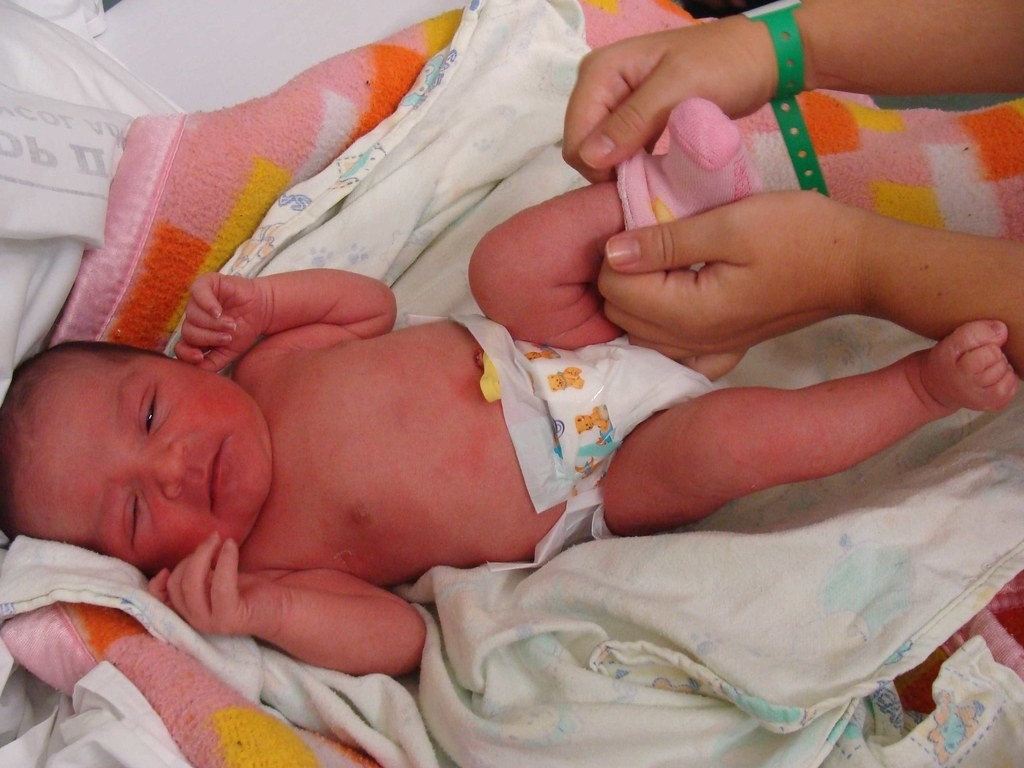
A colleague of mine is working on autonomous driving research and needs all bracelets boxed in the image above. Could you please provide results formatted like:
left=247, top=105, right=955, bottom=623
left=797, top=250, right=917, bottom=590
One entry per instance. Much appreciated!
left=741, top=0, right=829, bottom=200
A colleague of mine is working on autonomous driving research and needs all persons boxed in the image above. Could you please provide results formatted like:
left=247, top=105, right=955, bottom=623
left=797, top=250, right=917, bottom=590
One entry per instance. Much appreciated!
left=561, top=0, right=1023, bottom=376
left=3, top=97, right=1024, bottom=676
